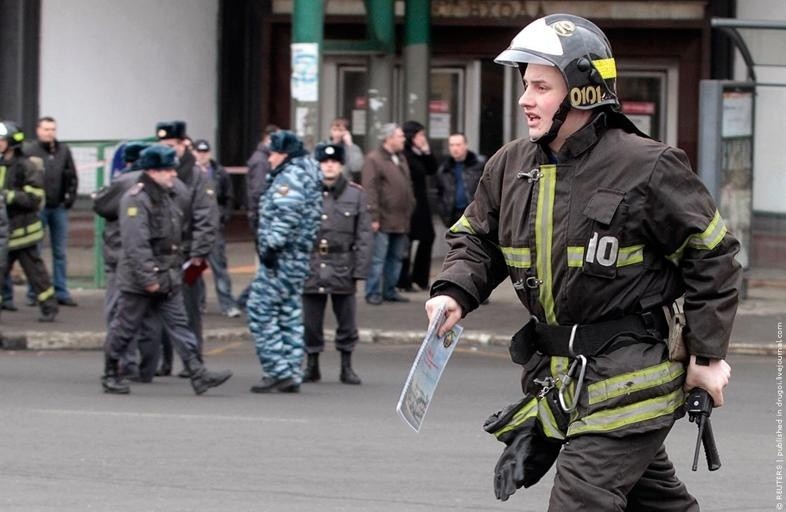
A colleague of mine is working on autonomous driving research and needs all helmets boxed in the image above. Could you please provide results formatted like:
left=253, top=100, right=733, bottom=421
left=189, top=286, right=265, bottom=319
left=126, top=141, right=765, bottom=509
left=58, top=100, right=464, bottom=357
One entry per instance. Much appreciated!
left=0, top=120, right=25, bottom=151
left=495, top=12, right=620, bottom=112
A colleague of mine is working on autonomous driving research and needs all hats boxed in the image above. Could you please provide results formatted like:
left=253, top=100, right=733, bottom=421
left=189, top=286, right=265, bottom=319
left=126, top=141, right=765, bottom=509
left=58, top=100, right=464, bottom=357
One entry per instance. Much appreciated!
left=403, top=119, right=422, bottom=139
left=122, top=120, right=346, bottom=168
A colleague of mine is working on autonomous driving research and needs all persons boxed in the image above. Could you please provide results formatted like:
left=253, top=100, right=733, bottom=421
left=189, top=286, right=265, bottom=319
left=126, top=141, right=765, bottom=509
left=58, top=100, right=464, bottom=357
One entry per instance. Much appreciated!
left=1, top=114, right=487, bottom=395
left=425, top=13, right=742, bottom=512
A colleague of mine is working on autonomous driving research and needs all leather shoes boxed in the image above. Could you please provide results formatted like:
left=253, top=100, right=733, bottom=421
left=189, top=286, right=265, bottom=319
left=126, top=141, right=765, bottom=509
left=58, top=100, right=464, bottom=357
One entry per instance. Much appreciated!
left=365, top=277, right=431, bottom=305
left=0, top=289, right=80, bottom=322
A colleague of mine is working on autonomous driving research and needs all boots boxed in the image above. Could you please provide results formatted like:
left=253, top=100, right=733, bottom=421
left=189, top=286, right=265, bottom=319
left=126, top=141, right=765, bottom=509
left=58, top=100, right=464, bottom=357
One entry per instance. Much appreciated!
left=98, top=305, right=364, bottom=398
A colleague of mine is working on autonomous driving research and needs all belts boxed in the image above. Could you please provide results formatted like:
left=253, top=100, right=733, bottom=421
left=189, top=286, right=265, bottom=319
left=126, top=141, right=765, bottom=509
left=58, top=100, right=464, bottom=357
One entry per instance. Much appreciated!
left=501, top=318, right=677, bottom=365
left=312, top=242, right=354, bottom=256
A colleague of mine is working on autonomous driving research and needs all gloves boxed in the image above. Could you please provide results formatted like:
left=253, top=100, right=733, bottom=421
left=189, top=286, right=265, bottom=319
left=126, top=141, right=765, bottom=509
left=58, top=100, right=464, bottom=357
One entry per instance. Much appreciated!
left=482, top=392, right=538, bottom=503
